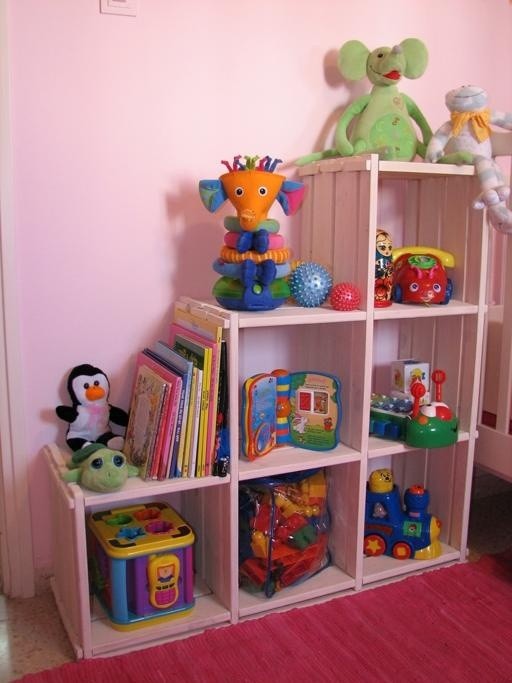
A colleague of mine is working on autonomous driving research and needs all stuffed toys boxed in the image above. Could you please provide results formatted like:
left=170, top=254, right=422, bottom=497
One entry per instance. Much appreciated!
left=56, top=364, right=129, bottom=452
left=426, top=84, right=512, bottom=235
left=293, top=37, right=473, bottom=167
left=60, top=442, right=139, bottom=492
left=199, top=153, right=308, bottom=310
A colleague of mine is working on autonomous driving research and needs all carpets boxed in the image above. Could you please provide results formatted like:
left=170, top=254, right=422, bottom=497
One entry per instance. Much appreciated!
left=19, top=550, right=512, bottom=683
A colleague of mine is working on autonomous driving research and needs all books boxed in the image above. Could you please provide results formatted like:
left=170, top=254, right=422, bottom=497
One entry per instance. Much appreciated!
left=121, top=294, right=230, bottom=481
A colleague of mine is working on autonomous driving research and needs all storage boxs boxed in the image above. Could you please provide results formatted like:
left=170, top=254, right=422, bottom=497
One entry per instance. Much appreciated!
left=391, top=357, right=431, bottom=405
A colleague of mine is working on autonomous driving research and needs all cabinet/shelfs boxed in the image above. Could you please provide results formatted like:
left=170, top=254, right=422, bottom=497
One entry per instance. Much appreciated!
left=83, top=478, right=229, bottom=639
left=362, top=159, right=490, bottom=583
left=233, top=314, right=356, bottom=608
